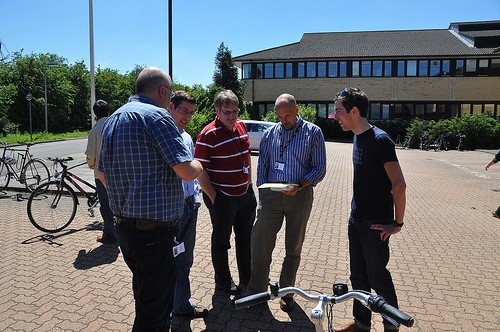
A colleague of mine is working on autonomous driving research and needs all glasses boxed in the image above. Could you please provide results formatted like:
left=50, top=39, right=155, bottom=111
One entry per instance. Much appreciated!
left=176, top=105, right=195, bottom=114
left=220, top=108, right=238, bottom=115
left=340, top=88, right=354, bottom=104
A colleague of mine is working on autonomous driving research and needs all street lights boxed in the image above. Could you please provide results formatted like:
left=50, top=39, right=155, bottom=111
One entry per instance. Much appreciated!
left=41, top=61, right=61, bottom=133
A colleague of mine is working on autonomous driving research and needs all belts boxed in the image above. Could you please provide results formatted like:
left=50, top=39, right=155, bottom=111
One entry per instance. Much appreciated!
left=112, top=215, right=179, bottom=231
left=185, top=194, right=194, bottom=205
left=274, top=182, right=288, bottom=183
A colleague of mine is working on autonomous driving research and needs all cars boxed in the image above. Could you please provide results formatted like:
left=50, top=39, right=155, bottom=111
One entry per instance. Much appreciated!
left=239, top=118, right=279, bottom=152
left=328, top=109, right=336, bottom=119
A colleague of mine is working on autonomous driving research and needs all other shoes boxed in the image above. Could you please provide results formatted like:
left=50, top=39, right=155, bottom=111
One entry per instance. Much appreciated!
left=97, top=238, right=104, bottom=243
left=335, top=323, right=370, bottom=332
left=384, top=328, right=399, bottom=332
left=280, top=293, right=295, bottom=312
left=179, top=307, right=208, bottom=319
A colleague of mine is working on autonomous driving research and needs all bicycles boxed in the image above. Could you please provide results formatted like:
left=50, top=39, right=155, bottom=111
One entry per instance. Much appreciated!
left=231, top=280, right=411, bottom=332
left=0, top=140, right=50, bottom=194
left=26, top=155, right=101, bottom=234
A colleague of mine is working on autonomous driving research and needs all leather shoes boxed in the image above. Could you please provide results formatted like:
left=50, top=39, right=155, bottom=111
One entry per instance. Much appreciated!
left=221, top=281, right=242, bottom=295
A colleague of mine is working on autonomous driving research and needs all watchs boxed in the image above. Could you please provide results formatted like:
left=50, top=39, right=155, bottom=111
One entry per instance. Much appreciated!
left=297, top=182, right=305, bottom=192
left=394, top=219, right=405, bottom=228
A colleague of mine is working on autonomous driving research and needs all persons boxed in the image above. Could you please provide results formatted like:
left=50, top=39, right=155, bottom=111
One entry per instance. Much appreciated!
left=484, top=150, right=500, bottom=218
left=334, top=87, right=407, bottom=332
left=86, top=100, right=119, bottom=245
left=167, top=90, right=210, bottom=321
left=192, top=88, right=257, bottom=295
left=97, top=66, right=204, bottom=332
left=246, top=94, right=327, bottom=311
